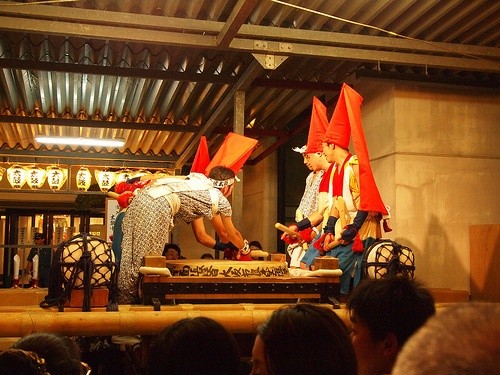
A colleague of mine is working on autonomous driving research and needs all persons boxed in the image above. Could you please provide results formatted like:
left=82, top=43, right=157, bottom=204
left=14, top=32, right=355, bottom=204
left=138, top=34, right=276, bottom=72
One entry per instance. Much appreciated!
left=26, top=233, right=54, bottom=287
left=0, top=280, right=500, bottom=375
left=108, top=166, right=250, bottom=304
left=162, top=241, right=264, bottom=262
left=282, top=131, right=382, bottom=307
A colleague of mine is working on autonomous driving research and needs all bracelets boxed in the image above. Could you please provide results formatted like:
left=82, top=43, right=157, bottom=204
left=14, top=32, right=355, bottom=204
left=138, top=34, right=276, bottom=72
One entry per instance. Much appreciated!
left=241, top=241, right=250, bottom=255
left=312, top=227, right=320, bottom=235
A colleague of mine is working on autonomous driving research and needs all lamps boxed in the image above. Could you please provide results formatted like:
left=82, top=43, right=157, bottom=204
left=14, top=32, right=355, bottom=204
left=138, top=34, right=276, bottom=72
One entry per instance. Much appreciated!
left=36, top=134, right=127, bottom=148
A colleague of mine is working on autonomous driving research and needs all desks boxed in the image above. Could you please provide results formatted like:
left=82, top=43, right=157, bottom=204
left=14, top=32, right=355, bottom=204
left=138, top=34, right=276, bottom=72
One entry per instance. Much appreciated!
left=139, top=254, right=342, bottom=308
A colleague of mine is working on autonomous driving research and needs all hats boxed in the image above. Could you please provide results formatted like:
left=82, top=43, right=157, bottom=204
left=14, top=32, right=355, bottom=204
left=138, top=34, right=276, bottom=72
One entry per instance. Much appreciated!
left=205, top=132, right=259, bottom=175
left=305, top=96, right=328, bottom=154
left=33, top=232, right=45, bottom=239
left=323, top=82, right=364, bottom=150
left=191, top=136, right=211, bottom=173
